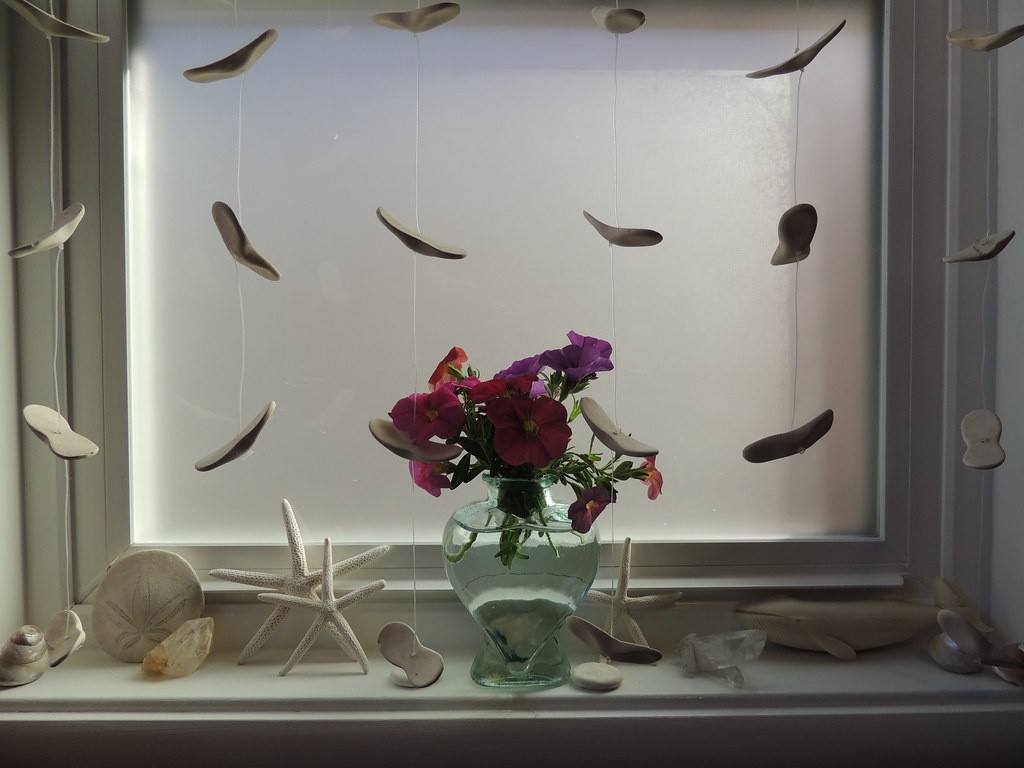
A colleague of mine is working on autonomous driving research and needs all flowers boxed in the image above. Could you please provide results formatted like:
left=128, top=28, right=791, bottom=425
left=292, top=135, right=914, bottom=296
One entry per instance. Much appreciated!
left=388, top=331, right=664, bottom=564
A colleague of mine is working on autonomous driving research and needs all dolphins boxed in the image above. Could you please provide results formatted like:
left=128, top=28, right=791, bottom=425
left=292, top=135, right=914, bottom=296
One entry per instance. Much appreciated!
left=735, top=601, right=994, bottom=661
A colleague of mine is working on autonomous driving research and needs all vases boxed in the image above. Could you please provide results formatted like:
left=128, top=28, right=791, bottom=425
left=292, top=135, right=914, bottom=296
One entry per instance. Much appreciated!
left=440, top=473, right=601, bottom=691
